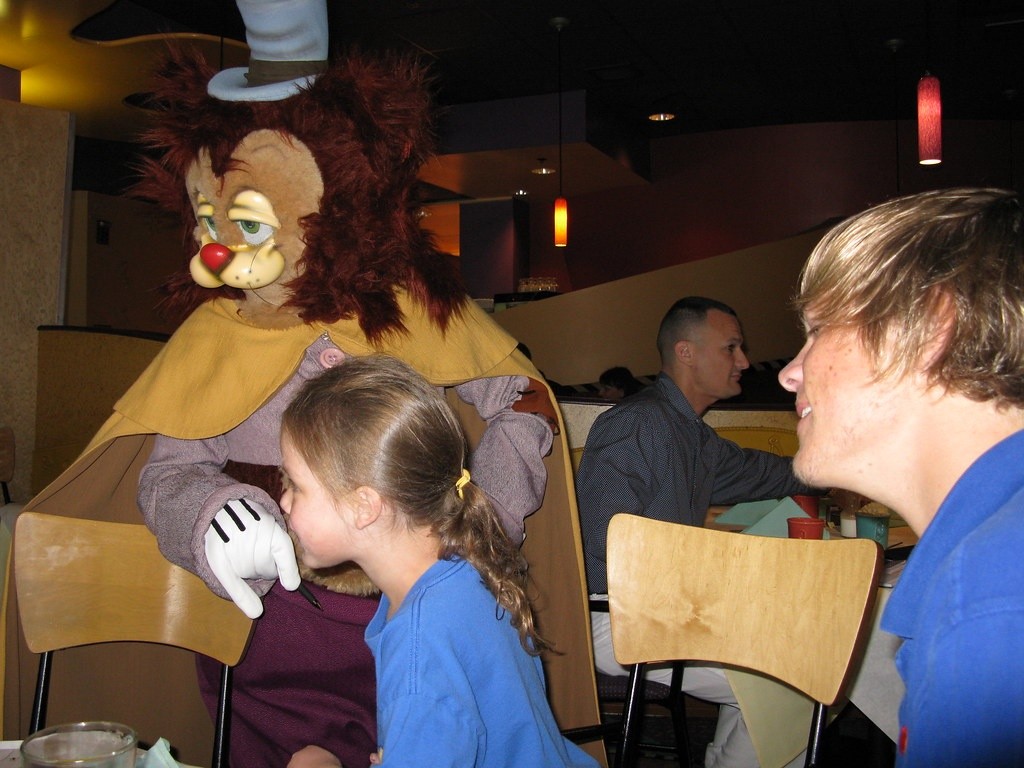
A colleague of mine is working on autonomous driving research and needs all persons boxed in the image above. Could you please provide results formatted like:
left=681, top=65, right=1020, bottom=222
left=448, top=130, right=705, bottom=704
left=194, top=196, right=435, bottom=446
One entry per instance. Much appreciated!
left=776, top=188, right=1024, bottom=768
left=575, top=296, right=832, bottom=768
left=518, top=343, right=570, bottom=396
left=279, top=355, right=601, bottom=768
left=598, top=366, right=637, bottom=401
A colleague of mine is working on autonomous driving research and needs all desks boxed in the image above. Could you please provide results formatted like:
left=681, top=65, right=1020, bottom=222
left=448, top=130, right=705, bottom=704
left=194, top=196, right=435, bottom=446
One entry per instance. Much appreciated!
left=712, top=516, right=919, bottom=763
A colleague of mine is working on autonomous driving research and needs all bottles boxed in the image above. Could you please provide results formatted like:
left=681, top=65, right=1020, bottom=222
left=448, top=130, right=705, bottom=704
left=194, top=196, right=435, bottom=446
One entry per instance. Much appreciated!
left=517, top=276, right=559, bottom=292
left=840, top=490, right=860, bottom=538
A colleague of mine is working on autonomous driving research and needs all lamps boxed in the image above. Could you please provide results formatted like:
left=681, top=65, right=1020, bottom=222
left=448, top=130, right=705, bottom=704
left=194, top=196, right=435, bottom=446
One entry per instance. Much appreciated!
left=917, top=67, right=943, bottom=165
left=554, top=81, right=567, bottom=248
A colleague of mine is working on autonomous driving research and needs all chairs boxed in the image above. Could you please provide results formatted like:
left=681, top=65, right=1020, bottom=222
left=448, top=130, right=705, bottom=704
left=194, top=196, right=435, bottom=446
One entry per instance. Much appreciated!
left=12, top=512, right=257, bottom=768
left=605, top=511, right=884, bottom=767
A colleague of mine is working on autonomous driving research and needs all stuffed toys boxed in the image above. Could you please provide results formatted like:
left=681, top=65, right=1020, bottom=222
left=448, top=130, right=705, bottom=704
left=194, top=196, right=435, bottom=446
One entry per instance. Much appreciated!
left=0, top=61, right=558, bottom=768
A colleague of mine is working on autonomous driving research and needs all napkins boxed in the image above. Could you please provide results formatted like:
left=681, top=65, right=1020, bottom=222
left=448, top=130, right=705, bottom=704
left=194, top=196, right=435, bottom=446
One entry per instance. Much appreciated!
left=713, top=492, right=831, bottom=541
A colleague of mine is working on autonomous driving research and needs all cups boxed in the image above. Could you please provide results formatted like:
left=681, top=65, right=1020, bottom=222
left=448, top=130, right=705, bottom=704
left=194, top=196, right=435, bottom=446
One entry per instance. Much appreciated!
left=20, top=721, right=138, bottom=768
left=792, top=494, right=819, bottom=519
left=855, top=511, right=891, bottom=551
left=787, top=517, right=826, bottom=540
